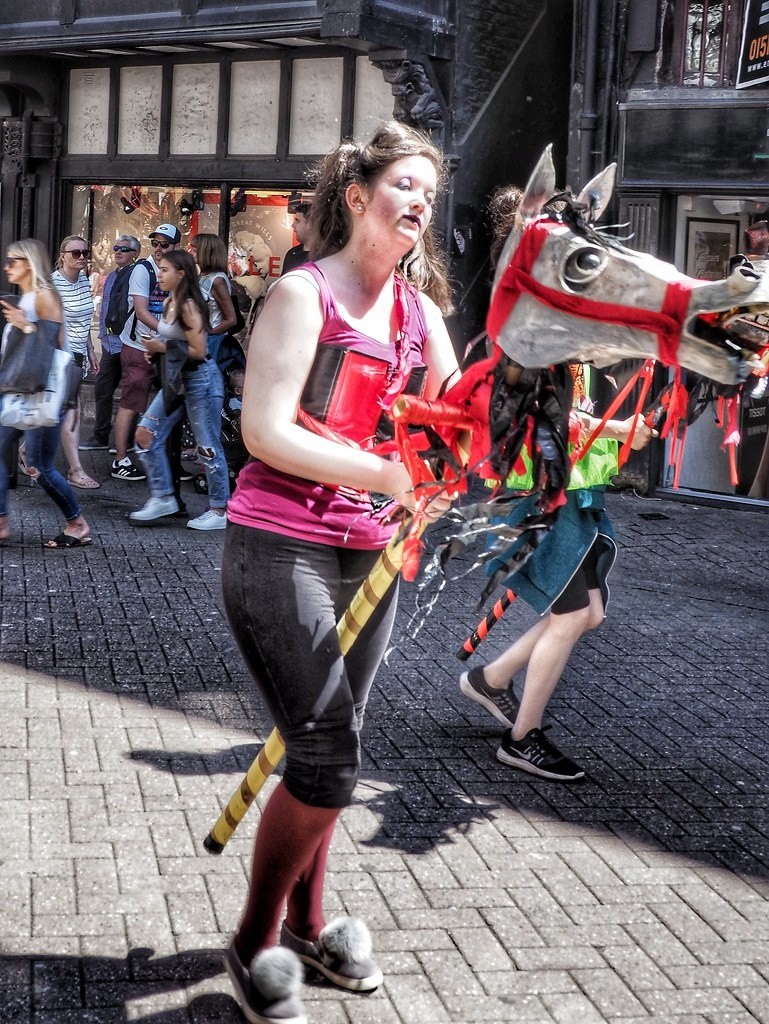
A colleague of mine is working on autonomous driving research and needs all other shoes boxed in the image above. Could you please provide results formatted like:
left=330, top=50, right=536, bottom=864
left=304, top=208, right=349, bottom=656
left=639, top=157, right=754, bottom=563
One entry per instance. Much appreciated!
left=280, top=915, right=383, bottom=992
left=18, top=447, right=28, bottom=475
left=224, top=938, right=310, bottom=1024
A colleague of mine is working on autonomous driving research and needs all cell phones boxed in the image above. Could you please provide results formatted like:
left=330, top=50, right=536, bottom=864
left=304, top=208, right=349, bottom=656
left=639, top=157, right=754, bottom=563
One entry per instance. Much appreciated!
left=1, top=295, right=17, bottom=310
left=141, top=334, right=151, bottom=340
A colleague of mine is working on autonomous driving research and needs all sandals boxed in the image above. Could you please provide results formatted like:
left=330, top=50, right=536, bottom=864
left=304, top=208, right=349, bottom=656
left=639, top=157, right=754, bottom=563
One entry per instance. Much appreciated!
left=67, top=468, right=100, bottom=489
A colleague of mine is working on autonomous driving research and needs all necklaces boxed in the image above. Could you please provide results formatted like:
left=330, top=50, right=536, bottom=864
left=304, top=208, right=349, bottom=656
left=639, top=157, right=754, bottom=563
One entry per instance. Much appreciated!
left=169, top=298, right=177, bottom=312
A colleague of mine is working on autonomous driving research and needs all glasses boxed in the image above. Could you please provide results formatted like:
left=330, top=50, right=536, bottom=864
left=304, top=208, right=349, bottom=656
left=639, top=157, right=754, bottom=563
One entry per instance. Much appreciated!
left=113, top=244, right=136, bottom=252
left=4, top=255, right=27, bottom=268
left=150, top=239, right=169, bottom=249
left=62, top=249, right=89, bottom=258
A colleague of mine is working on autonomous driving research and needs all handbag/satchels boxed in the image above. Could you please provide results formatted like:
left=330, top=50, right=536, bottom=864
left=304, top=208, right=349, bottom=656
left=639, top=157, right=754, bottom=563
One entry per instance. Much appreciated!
left=0, top=319, right=63, bottom=394
left=0, top=348, right=72, bottom=430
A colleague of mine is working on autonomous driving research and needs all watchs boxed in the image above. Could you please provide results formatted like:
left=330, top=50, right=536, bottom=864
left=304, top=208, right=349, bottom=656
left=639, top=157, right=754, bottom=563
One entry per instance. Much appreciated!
left=22, top=321, right=33, bottom=336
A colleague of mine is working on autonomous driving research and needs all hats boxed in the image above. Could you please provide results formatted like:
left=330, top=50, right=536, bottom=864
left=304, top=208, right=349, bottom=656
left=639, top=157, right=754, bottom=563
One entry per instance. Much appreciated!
left=148, top=224, right=181, bottom=244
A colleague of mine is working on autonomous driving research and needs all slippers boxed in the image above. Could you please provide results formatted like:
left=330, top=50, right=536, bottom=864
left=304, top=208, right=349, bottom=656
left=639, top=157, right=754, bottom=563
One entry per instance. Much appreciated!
left=44, top=532, right=93, bottom=547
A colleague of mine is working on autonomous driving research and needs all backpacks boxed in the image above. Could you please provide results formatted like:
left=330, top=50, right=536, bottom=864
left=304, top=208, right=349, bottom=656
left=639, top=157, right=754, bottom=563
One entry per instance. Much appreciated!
left=105, top=258, right=157, bottom=341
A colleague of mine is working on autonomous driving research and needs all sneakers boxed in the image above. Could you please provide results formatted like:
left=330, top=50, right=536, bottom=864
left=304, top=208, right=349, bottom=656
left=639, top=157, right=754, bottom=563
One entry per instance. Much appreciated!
left=460, top=666, right=519, bottom=727
left=187, top=510, right=227, bottom=530
left=130, top=497, right=178, bottom=520
left=110, top=457, right=146, bottom=481
left=496, top=729, right=585, bottom=782
left=78, top=435, right=109, bottom=450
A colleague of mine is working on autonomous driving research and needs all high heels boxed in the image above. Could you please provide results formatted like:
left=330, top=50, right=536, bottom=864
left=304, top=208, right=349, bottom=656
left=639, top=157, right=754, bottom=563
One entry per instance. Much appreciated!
left=180, top=464, right=193, bottom=480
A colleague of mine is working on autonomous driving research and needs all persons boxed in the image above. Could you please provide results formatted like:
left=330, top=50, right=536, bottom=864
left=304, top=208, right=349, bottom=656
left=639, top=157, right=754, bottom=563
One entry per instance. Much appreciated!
left=129, top=250, right=229, bottom=530
left=459, top=190, right=604, bottom=781
left=221, top=122, right=463, bottom=1024
left=281, top=203, right=311, bottom=275
left=77, top=235, right=140, bottom=453
left=0, top=239, right=92, bottom=547
left=18, top=235, right=100, bottom=488
left=111, top=223, right=181, bottom=480
left=180, top=233, right=237, bottom=459
